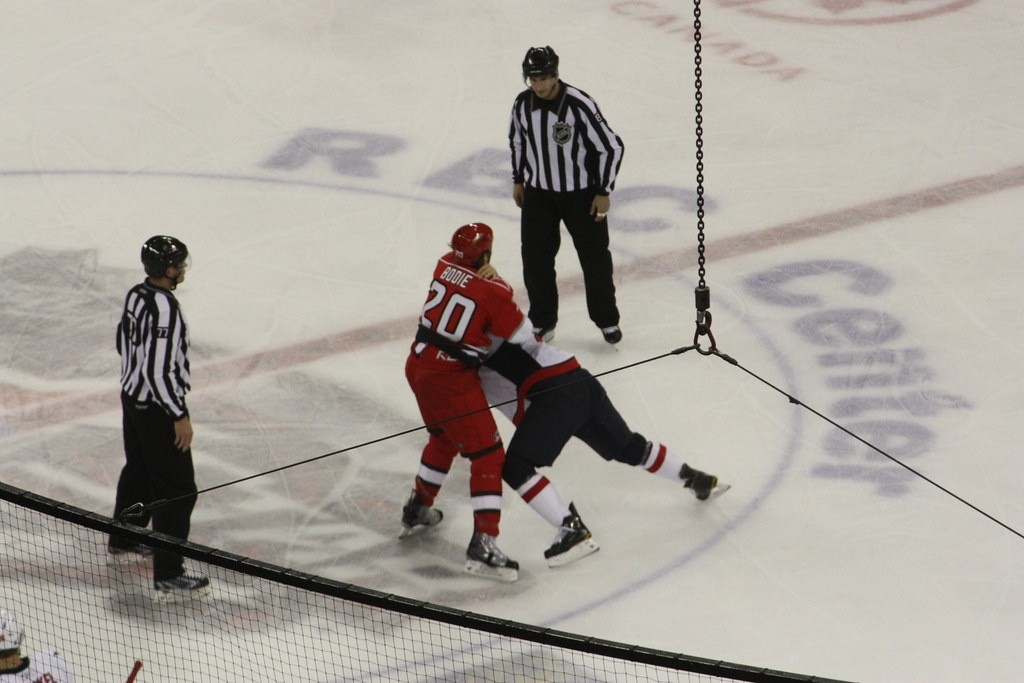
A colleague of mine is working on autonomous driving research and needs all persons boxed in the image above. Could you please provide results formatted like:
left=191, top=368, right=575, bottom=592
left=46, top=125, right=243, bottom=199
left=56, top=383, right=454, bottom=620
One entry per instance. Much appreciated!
left=508, top=46, right=624, bottom=353
left=398, top=224, right=732, bottom=583
left=107, top=235, right=210, bottom=590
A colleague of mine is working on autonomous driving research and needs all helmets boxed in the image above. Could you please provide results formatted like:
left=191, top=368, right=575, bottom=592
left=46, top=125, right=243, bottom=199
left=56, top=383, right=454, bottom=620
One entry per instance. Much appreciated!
left=522, top=46, right=559, bottom=76
left=0, top=609, right=23, bottom=651
left=452, top=223, right=493, bottom=266
left=141, top=235, right=188, bottom=279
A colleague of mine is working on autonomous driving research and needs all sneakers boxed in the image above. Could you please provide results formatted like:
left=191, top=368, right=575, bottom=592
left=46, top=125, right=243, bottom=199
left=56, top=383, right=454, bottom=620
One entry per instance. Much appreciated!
left=106, top=542, right=154, bottom=564
left=679, top=463, right=731, bottom=503
left=602, top=325, right=622, bottom=352
left=544, top=501, right=600, bottom=568
left=155, top=572, right=211, bottom=603
left=398, top=489, right=444, bottom=539
left=463, top=533, right=519, bottom=582
left=532, top=327, right=555, bottom=347
